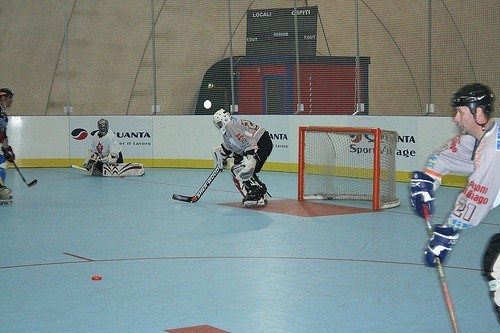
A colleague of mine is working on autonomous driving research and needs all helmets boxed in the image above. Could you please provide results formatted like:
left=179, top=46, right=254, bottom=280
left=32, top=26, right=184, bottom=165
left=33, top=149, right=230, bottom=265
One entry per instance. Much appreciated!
left=450, top=83, right=496, bottom=114
left=213, top=109, right=231, bottom=135
left=0, top=88, right=14, bottom=97
left=97, top=118, right=109, bottom=126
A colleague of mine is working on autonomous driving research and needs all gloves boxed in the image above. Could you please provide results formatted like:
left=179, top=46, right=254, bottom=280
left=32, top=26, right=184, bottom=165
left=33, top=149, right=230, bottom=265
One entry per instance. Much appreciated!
left=3, top=146, right=16, bottom=163
left=425, top=225, right=460, bottom=268
left=411, top=171, right=437, bottom=217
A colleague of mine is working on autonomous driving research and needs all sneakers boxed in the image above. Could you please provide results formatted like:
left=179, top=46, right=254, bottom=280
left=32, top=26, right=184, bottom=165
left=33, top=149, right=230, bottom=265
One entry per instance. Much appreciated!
left=243, top=194, right=270, bottom=208
left=0, top=187, right=14, bottom=205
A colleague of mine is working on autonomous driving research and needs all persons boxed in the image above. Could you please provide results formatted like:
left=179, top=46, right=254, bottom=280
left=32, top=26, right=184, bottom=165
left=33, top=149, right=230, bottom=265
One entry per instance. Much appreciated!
left=0, top=87, right=15, bottom=205
left=411, top=84, right=499, bottom=323
left=91, top=119, right=123, bottom=175
left=212, top=109, right=272, bottom=208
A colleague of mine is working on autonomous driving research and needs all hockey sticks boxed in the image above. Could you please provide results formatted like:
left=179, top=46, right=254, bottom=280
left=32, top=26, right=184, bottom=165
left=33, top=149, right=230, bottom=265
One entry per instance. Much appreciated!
left=11, top=157, right=38, bottom=188
left=422, top=199, right=458, bottom=333
left=172, top=156, right=227, bottom=203
